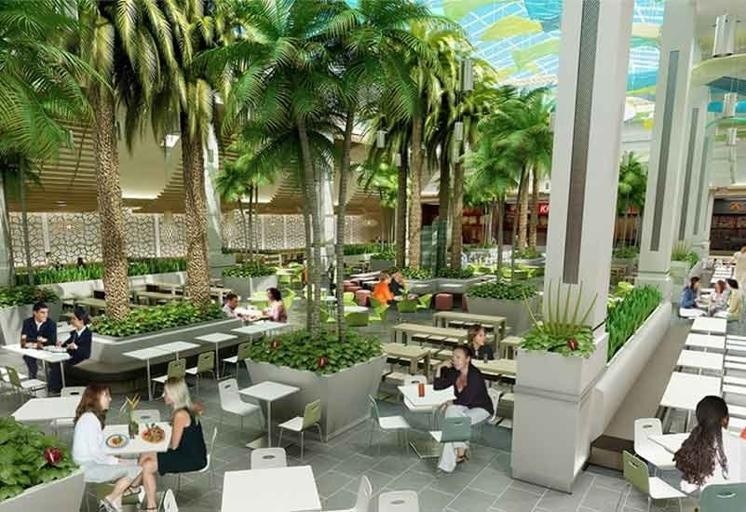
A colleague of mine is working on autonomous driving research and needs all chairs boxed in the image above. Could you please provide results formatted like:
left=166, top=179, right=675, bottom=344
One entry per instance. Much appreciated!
left=622, top=451, right=687, bottom=511
left=379, top=489, right=420, bottom=511
left=697, top=483, right=746, bottom=512
left=186, top=351, right=217, bottom=396
left=276, top=398, right=324, bottom=463
left=6, top=366, right=48, bottom=397
left=151, top=359, right=186, bottom=396
left=250, top=446, right=286, bottom=469
left=634, top=418, right=677, bottom=477
left=221, top=342, right=251, bottom=379
left=343, top=475, right=373, bottom=512
left=368, top=394, right=412, bottom=457
left=276, top=260, right=339, bottom=319
left=344, top=289, right=432, bottom=327
left=721, top=330, right=746, bottom=435
left=130, top=407, right=161, bottom=422
left=217, top=378, right=262, bottom=440
left=51, top=386, right=87, bottom=428
left=166, top=428, right=219, bottom=493
left=428, top=417, right=471, bottom=465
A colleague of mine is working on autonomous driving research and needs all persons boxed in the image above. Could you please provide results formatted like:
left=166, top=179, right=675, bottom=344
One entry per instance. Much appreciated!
left=221, top=294, right=247, bottom=323
left=677, top=277, right=701, bottom=317
left=50, top=311, right=92, bottom=388
left=375, top=268, right=407, bottom=308
left=465, top=325, right=494, bottom=360
left=675, top=396, right=746, bottom=494
left=263, top=289, right=288, bottom=323
left=434, top=344, right=493, bottom=472
left=727, top=246, right=745, bottom=287
left=19, top=302, right=58, bottom=382
left=71, top=383, right=141, bottom=510
left=712, top=280, right=742, bottom=319
left=123, top=378, right=206, bottom=510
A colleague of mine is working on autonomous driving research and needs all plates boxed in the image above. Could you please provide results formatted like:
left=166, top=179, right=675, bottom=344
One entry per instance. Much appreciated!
left=105, top=434, right=129, bottom=448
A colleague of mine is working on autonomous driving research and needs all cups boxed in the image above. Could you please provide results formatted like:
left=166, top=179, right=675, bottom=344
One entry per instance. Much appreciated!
left=418, top=383, right=425, bottom=397
left=21, top=335, right=26, bottom=348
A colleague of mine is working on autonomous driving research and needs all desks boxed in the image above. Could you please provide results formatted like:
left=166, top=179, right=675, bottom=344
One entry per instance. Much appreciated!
left=659, top=371, right=721, bottom=433
left=685, top=332, right=726, bottom=352
left=4, top=345, right=72, bottom=386
left=649, top=432, right=693, bottom=457
left=398, top=382, right=457, bottom=459
left=159, top=341, right=201, bottom=359
left=221, top=465, right=322, bottom=512
left=691, top=316, right=727, bottom=335
left=194, top=332, right=239, bottom=381
left=12, top=396, right=86, bottom=436
left=381, top=344, right=433, bottom=375
left=65, top=277, right=231, bottom=309
left=390, top=324, right=469, bottom=350
left=677, top=349, right=723, bottom=376
left=121, top=346, right=171, bottom=400
left=237, top=381, right=301, bottom=447
left=232, top=324, right=268, bottom=345
left=106, top=422, right=171, bottom=456
left=434, top=311, right=508, bottom=354
left=697, top=262, right=734, bottom=313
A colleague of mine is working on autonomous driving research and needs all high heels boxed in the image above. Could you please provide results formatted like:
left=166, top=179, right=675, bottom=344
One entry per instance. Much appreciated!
left=460, top=449, right=470, bottom=460
left=98, top=496, right=119, bottom=512
left=456, top=458, right=464, bottom=471
